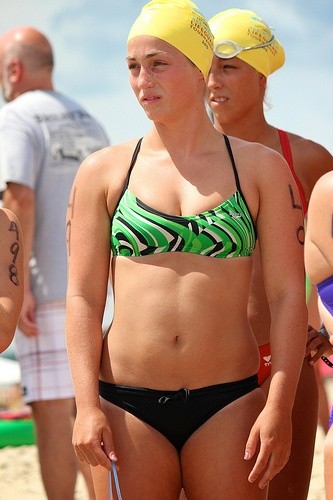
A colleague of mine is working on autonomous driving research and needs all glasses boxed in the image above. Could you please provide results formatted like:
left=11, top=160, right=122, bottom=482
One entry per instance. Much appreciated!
left=214, top=35, right=276, bottom=59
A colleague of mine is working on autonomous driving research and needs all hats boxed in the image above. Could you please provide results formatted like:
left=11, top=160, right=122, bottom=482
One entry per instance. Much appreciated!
left=208, top=8, right=285, bottom=77
left=126, top=0, right=212, bottom=84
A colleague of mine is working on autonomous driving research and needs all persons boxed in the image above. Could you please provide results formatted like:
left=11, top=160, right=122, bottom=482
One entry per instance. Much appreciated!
left=61, top=0, right=308, bottom=500
left=0, top=24, right=115, bottom=500
left=196, top=3, right=333, bottom=500
